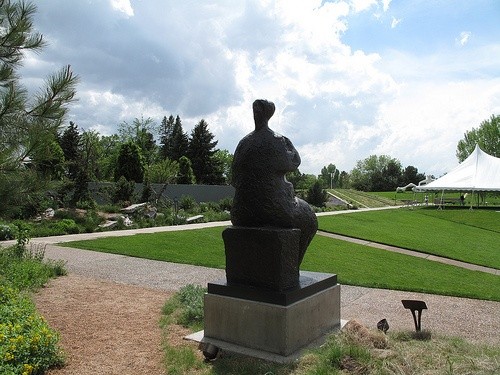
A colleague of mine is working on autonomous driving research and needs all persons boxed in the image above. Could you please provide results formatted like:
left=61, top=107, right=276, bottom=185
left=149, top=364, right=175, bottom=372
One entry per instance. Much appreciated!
left=461, top=194, right=464, bottom=204
left=425, top=194, right=428, bottom=205
left=231, top=99, right=319, bottom=268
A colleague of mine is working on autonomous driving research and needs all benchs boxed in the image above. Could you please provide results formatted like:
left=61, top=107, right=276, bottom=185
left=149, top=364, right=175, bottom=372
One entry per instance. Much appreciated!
left=435, top=196, right=466, bottom=204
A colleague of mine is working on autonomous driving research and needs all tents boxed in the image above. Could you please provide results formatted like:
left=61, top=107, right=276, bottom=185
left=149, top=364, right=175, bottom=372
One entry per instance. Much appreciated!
left=412, top=144, right=500, bottom=213
